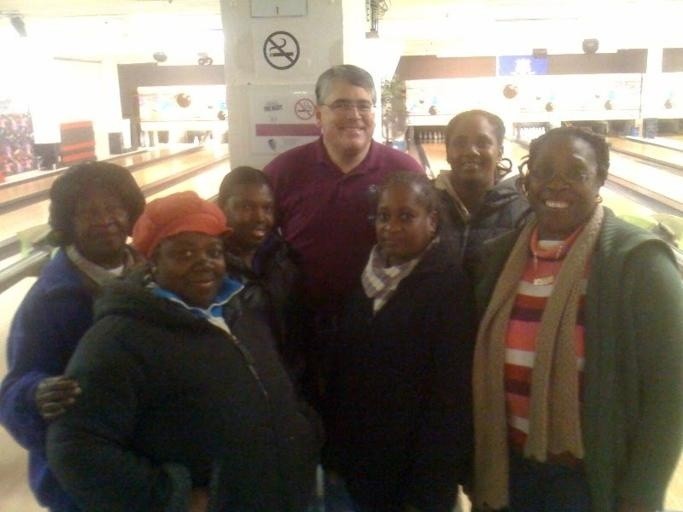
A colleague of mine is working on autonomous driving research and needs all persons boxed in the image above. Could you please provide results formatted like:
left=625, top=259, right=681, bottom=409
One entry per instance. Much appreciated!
left=261, top=64, right=428, bottom=512
left=0, top=162, right=146, bottom=512
left=459, top=127, right=683, bottom=512
left=430, top=109, right=521, bottom=232
left=46, top=191, right=321, bottom=512
left=318, top=172, right=463, bottom=512
left=218, top=167, right=333, bottom=430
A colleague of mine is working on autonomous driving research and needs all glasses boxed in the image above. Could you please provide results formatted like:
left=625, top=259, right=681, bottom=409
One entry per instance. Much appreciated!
left=323, top=102, right=375, bottom=115
left=528, top=164, right=597, bottom=183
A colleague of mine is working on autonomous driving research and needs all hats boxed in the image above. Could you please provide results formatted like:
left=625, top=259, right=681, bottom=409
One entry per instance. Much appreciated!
left=132, top=192, right=233, bottom=259
